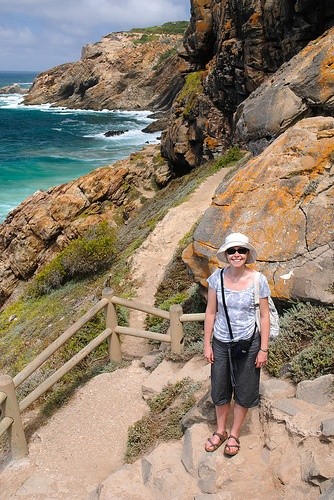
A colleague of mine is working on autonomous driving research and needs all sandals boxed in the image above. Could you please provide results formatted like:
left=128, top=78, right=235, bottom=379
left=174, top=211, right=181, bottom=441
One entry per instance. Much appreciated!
left=205, top=431, right=228, bottom=451
left=224, top=436, right=241, bottom=456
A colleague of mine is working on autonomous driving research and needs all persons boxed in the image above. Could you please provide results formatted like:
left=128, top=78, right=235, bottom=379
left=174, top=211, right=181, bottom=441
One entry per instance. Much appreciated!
left=202, top=233, right=271, bottom=456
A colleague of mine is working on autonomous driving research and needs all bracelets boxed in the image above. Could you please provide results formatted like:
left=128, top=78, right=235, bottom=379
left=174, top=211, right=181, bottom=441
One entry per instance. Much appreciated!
left=260, top=349, right=268, bottom=352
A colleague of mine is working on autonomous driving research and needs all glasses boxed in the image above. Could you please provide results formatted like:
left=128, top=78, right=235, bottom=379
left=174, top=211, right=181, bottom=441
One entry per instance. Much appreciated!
left=226, top=248, right=247, bottom=254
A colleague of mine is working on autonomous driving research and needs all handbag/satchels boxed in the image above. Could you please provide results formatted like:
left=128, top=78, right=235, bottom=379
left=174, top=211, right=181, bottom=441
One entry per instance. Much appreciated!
left=255, top=272, right=280, bottom=338
left=232, top=340, right=251, bottom=359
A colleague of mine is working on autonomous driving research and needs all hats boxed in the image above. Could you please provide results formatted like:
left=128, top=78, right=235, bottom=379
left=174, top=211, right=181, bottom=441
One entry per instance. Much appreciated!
left=217, top=232, right=257, bottom=265
